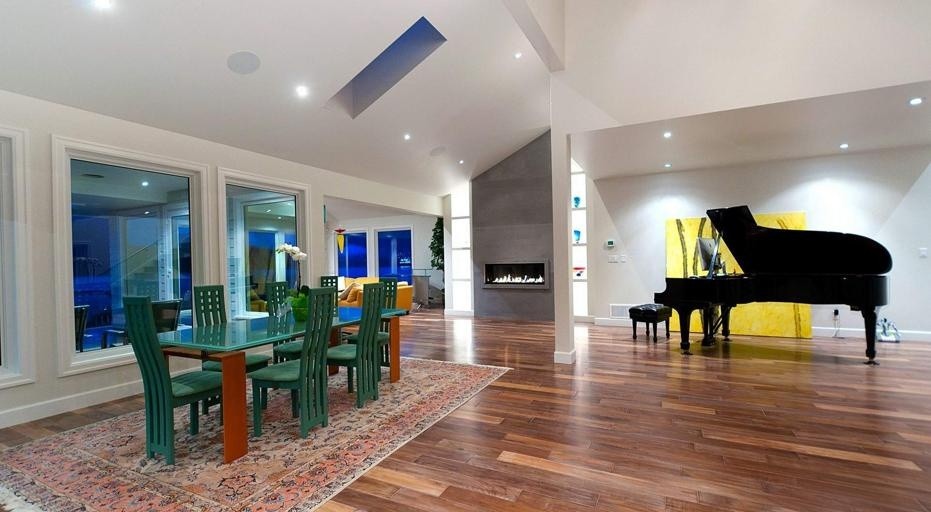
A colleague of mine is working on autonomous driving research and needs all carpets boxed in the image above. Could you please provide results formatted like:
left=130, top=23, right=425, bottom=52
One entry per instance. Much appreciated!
left=2, top=352, right=514, bottom=512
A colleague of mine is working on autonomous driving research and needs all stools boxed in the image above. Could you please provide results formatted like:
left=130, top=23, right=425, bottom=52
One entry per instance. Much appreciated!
left=629, top=304, right=672, bottom=342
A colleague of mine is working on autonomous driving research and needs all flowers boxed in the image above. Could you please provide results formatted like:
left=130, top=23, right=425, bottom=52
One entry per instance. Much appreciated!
left=276, top=243, right=307, bottom=299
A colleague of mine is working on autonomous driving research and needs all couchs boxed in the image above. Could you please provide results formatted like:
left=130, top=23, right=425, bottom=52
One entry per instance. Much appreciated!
left=338, top=285, right=414, bottom=315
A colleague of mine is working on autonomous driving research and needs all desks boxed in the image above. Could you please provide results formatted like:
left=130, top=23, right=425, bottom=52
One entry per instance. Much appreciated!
left=155, top=306, right=409, bottom=464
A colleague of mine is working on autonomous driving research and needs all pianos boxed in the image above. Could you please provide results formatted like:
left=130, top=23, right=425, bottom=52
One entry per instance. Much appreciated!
left=654, top=205, right=892, bottom=365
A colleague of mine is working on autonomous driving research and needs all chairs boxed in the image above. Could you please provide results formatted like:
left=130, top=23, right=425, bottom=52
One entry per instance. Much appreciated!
left=346, top=281, right=398, bottom=381
left=194, top=284, right=272, bottom=414
left=122, top=297, right=223, bottom=463
left=247, top=287, right=336, bottom=440
left=316, top=284, right=386, bottom=409
left=74, top=298, right=182, bottom=352
left=266, top=282, right=296, bottom=395
